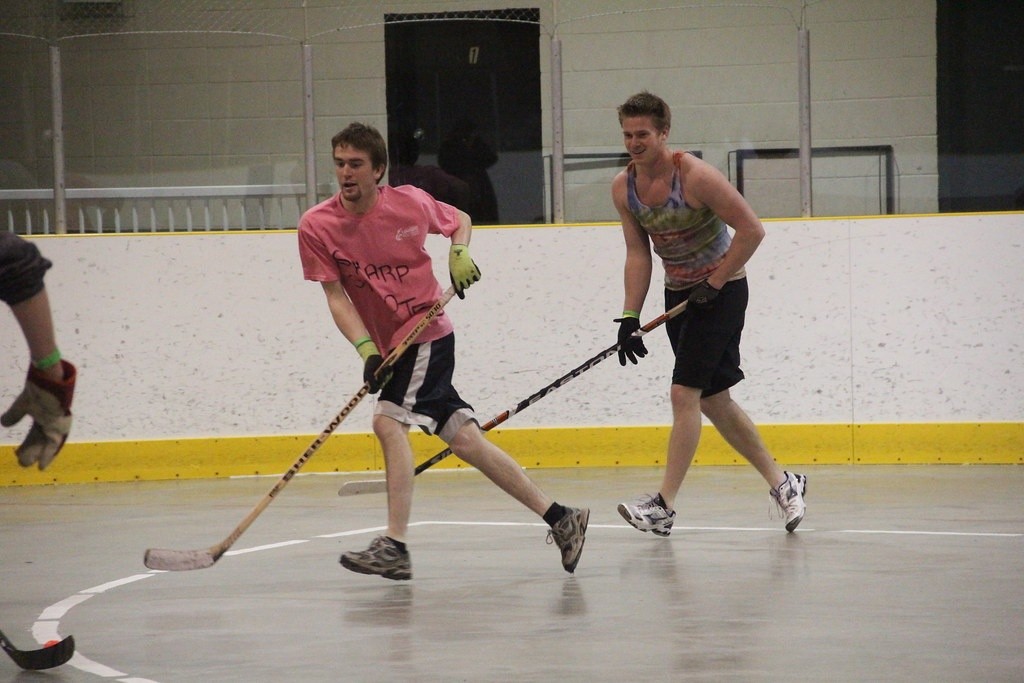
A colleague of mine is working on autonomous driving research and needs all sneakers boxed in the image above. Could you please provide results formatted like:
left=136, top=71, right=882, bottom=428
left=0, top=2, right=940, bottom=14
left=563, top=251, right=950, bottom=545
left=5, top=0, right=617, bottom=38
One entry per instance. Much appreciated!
left=618, top=493, right=676, bottom=536
left=769, top=470, right=807, bottom=533
left=545, top=506, right=590, bottom=573
left=341, top=534, right=412, bottom=580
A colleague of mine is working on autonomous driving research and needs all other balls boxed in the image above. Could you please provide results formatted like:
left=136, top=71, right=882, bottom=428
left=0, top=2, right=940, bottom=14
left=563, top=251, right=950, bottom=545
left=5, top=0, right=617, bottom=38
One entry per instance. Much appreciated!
left=44, top=639, right=60, bottom=648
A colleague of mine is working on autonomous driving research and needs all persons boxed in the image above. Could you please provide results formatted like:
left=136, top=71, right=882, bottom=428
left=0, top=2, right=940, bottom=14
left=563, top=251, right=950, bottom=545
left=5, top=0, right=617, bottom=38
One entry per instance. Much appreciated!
left=389, top=132, right=471, bottom=210
left=612, top=94, right=807, bottom=536
left=0, top=227, right=78, bottom=472
left=297, top=122, right=590, bottom=580
left=437, top=121, right=499, bottom=225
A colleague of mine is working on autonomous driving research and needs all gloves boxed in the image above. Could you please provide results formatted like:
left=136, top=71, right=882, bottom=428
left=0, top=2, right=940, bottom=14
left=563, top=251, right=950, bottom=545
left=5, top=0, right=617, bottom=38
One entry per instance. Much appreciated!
left=1, top=359, right=75, bottom=469
left=449, top=243, right=481, bottom=299
left=354, top=340, right=393, bottom=394
left=686, top=279, right=720, bottom=321
left=613, top=310, right=649, bottom=365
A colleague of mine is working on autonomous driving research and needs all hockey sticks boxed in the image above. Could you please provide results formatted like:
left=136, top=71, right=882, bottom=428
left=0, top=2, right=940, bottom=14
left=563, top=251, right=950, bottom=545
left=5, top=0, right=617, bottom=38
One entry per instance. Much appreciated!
left=339, top=300, right=688, bottom=494
left=0, top=630, right=76, bottom=672
left=145, top=284, right=457, bottom=571
